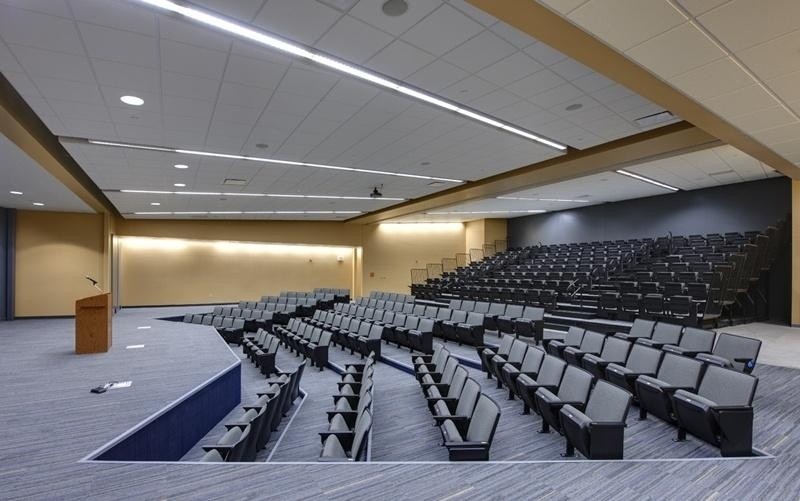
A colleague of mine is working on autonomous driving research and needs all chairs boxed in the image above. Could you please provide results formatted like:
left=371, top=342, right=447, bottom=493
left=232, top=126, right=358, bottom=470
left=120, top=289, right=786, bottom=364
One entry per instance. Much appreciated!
left=182, top=232, right=781, bottom=464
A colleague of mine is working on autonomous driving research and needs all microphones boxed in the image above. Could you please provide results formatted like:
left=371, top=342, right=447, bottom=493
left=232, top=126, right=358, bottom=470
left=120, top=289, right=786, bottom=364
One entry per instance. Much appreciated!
left=86, top=276, right=98, bottom=283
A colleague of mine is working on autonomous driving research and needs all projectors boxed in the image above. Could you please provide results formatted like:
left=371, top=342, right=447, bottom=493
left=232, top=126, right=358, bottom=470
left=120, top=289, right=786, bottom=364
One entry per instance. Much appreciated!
left=370, top=193, right=382, bottom=197
